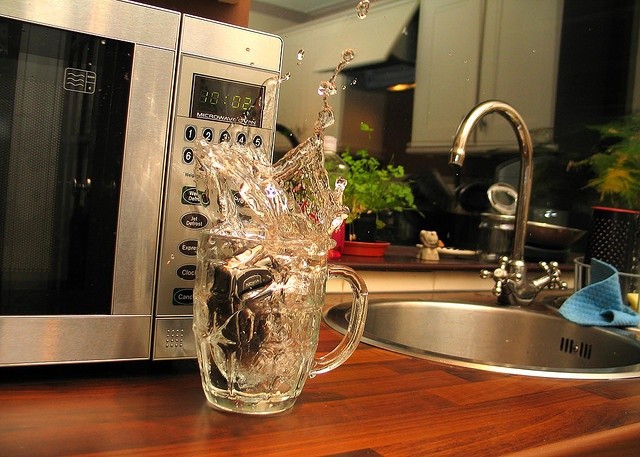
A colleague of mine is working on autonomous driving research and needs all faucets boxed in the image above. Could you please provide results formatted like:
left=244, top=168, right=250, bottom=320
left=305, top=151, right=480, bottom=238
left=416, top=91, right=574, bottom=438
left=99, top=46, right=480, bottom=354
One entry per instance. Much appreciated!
left=448, top=100, right=567, bottom=306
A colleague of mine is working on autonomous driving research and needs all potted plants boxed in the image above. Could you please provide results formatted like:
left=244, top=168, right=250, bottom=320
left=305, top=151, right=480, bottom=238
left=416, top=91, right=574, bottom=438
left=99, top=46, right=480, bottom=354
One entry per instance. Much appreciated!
left=566, top=110, right=639, bottom=305
left=295, top=122, right=426, bottom=258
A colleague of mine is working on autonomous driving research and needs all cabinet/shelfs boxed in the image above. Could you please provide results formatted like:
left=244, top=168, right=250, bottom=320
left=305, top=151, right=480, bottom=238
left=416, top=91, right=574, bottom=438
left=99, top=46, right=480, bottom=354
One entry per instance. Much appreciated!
left=405, top=0, right=567, bottom=155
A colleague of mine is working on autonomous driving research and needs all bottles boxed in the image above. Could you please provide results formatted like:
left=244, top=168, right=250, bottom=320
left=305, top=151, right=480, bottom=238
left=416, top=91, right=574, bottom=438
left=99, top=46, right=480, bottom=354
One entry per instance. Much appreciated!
left=316, top=130, right=350, bottom=261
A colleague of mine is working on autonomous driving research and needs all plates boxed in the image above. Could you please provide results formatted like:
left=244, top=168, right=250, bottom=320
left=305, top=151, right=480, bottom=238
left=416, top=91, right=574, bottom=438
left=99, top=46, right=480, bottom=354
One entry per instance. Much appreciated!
left=342, top=239, right=391, bottom=257
left=415, top=240, right=483, bottom=260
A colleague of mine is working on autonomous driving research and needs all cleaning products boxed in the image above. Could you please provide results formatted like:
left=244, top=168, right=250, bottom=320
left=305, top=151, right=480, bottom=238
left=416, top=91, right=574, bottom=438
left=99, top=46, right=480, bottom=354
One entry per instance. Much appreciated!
left=303, top=136, right=348, bottom=259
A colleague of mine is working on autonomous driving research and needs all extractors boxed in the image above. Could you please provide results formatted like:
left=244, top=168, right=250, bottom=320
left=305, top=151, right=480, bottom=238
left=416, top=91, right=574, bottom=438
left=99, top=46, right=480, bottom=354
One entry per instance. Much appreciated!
left=326, top=15, right=422, bottom=94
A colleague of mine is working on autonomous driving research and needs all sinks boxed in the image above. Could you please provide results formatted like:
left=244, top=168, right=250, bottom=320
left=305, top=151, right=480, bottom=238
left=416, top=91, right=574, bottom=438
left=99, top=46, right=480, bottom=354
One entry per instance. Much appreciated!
left=324, top=302, right=639, bottom=402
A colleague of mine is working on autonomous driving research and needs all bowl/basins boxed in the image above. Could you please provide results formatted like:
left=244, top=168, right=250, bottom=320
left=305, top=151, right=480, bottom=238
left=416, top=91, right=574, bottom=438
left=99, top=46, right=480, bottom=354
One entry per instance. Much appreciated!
left=527, top=220, right=585, bottom=249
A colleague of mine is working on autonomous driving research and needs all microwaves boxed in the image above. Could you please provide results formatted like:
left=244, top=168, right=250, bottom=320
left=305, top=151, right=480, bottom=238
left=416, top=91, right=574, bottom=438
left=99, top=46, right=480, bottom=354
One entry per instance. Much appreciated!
left=1, top=0, right=291, bottom=377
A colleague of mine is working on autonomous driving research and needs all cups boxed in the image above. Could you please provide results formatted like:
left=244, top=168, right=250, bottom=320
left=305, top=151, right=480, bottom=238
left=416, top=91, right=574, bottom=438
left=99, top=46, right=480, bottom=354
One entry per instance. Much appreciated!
left=191, top=228, right=369, bottom=417
